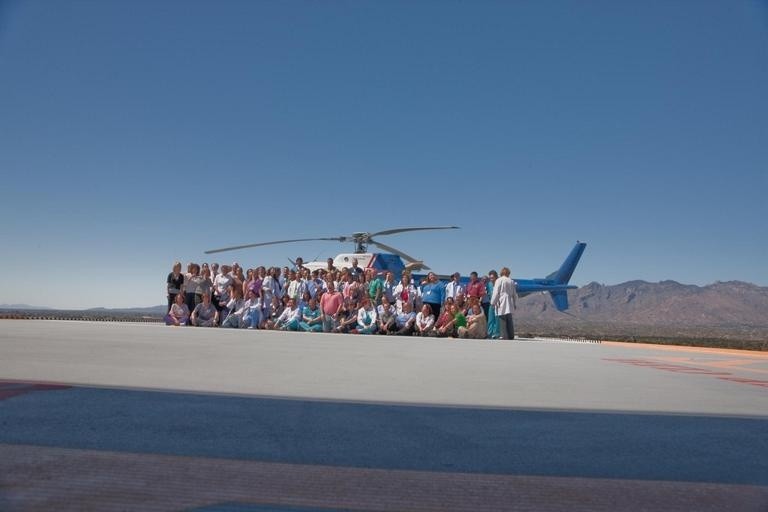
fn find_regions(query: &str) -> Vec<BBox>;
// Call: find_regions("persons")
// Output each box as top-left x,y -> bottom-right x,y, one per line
163,257 -> 516,340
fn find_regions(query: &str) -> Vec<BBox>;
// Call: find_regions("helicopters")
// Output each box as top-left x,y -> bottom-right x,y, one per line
204,225 -> 587,314
